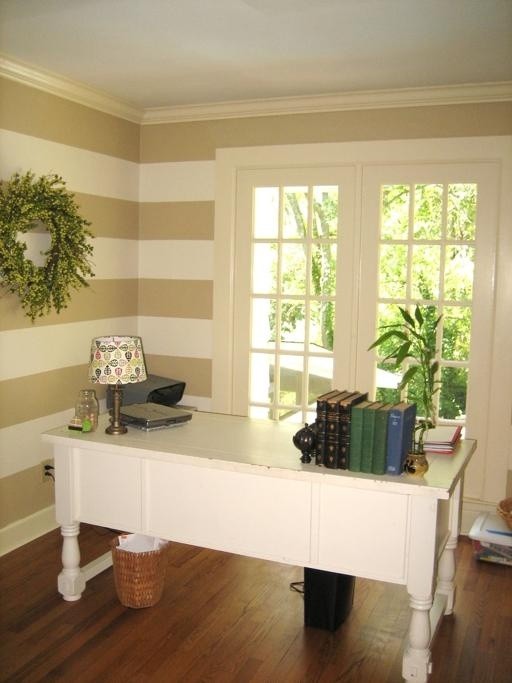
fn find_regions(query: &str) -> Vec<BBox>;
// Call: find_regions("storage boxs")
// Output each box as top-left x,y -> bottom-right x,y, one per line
468,512 -> 512,567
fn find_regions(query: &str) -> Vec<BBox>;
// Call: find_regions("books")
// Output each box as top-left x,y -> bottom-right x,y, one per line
313,388 -> 418,477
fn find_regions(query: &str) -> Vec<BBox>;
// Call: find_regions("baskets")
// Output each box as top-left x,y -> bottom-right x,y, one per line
112,536 -> 164,608
496,498 -> 512,530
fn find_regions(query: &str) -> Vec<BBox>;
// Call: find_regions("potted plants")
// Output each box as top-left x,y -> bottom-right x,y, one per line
365,304 -> 444,477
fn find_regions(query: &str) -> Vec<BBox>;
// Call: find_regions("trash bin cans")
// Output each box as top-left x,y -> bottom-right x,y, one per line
107,532 -> 169,610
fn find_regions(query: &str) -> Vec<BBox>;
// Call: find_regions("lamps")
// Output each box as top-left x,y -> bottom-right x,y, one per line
87,336 -> 147,436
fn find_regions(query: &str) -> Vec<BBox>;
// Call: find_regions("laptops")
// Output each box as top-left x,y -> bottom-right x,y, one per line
108,397 -> 193,429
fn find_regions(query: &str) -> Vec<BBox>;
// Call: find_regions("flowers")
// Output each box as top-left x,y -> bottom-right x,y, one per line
0,170 -> 96,326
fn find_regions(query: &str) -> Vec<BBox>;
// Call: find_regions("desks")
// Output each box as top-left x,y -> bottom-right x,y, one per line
39,403 -> 477,683
